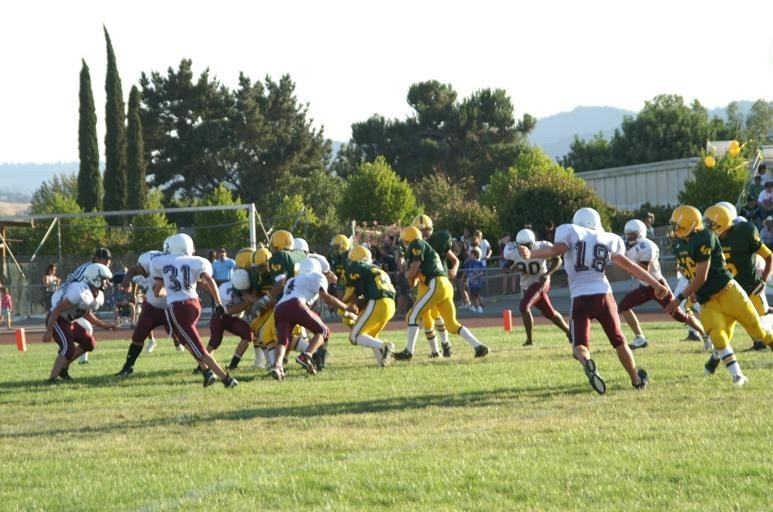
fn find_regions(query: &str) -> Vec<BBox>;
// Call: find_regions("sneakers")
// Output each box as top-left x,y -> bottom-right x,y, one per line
632,369 -> 648,392
629,338 -> 648,349
47,371 -> 71,384
731,375 -> 748,387
457,302 -> 483,313
680,331 -> 701,342
145,340 -> 156,352
176,344 -> 183,353
192,347 -> 326,388
375,328 -> 488,365
115,366 -> 132,377
567,334 -> 573,342
584,359 -> 606,395
746,342 -> 768,351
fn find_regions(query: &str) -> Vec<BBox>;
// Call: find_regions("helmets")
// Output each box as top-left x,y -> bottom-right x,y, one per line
348,246 -> 373,264
670,201 -> 739,240
515,228 -> 536,248
228,228 -> 330,293
624,219 -> 648,245
330,234 -> 349,251
399,226 -> 422,241
162,233 -> 194,257
83,263 -> 113,290
412,214 -> 433,230
572,207 -> 602,229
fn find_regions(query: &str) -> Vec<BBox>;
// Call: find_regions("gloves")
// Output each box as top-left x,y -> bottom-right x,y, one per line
213,305 -> 227,321
251,295 -> 271,313
750,279 -> 767,298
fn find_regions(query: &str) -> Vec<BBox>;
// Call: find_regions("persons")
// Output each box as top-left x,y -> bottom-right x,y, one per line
408,214 -> 460,358
392,226 -> 488,360
447,226 -> 491,312
642,162 -> 773,391
497,220 -> 557,295
51,248 -> 111,365
618,218 -> 711,350
519,207 -> 669,395
0,288 -> 12,329
333,217 -> 406,366
39,262 -> 117,387
150,233 -> 239,389
502,228 -> 572,346
112,229 -> 361,381
43,263 -> 61,327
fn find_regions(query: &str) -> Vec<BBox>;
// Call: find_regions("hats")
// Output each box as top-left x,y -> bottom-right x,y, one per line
746,195 -> 757,202
761,215 -> 773,227
95,247 -> 112,260
